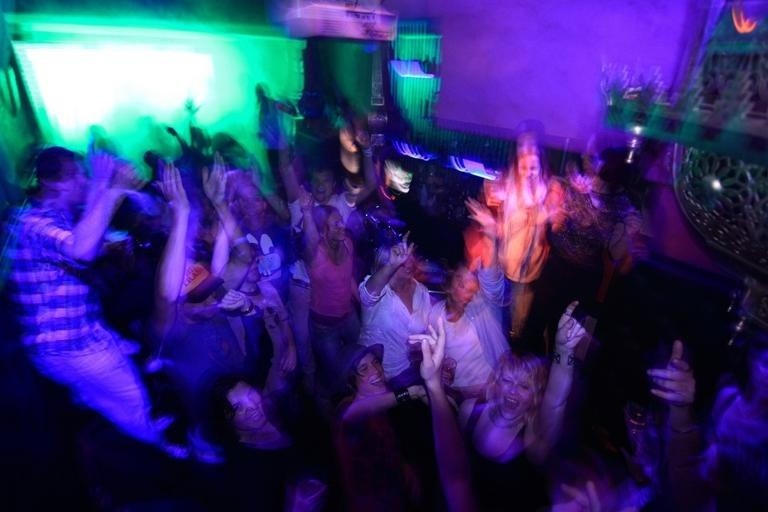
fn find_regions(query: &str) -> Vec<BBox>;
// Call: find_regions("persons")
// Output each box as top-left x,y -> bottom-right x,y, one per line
252,84 -> 296,191
0,118 -> 767,511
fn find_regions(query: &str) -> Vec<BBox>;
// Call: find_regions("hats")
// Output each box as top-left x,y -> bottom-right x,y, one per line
343,343 -> 384,374
177,257 -> 226,304
